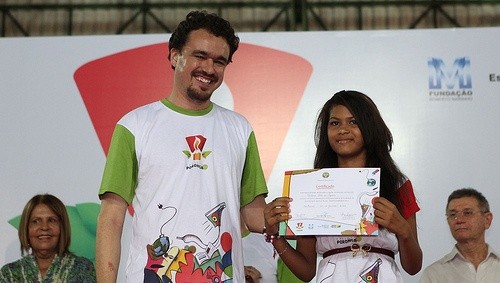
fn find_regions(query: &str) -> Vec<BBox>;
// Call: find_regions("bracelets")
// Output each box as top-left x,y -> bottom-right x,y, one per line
273,239 -> 288,259
263,226 -> 281,242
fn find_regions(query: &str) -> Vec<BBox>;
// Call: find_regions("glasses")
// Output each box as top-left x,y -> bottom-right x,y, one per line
445,210 -> 489,219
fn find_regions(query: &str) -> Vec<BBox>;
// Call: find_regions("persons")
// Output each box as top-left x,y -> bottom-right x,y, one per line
0,192 -> 97,283
418,188 -> 500,283
97,10 -> 269,283
263,91 -> 422,283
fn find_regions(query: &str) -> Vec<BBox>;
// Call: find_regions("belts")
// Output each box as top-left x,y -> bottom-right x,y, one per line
323,244 -> 394,260
